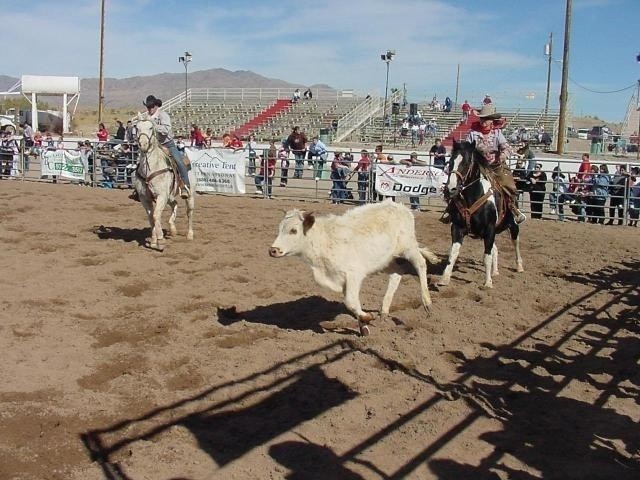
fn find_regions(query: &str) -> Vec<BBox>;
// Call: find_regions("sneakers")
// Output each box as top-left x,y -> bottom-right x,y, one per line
280,182 -> 287,186
255,190 -> 263,195
180,183 -> 191,200
550,211 -> 556,215
315,177 -> 320,181
307,165 -> 314,169
512,209 -> 525,224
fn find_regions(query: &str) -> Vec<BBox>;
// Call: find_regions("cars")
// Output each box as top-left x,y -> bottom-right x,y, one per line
568,124 -> 615,142
87,139 -> 137,178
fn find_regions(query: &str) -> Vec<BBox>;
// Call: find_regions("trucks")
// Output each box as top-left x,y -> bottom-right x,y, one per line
4,108 -> 72,133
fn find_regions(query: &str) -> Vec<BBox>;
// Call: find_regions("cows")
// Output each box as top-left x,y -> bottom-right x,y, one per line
268,196 -> 443,337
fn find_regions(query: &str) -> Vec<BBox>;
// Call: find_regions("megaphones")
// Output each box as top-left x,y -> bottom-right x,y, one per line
381,49 -> 395,61
179,51 -> 192,62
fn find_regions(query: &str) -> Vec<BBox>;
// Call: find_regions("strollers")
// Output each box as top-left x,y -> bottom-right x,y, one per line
329,167 -> 356,203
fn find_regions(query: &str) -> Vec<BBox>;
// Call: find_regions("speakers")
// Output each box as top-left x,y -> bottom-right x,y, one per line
392,103 -> 400,114
410,103 -> 417,115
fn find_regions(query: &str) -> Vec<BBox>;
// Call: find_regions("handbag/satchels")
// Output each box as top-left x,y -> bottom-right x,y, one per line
515,177 -> 534,192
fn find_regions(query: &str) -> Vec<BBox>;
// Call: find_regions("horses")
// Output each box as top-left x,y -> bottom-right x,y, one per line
437,136 -> 524,293
129,111 -> 197,251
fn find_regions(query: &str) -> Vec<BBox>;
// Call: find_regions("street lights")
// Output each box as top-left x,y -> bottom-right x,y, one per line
178,51 -> 192,136
379,49 -> 396,150
544,30 -> 554,115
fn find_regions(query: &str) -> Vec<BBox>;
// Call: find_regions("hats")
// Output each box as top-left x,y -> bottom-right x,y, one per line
123,140 -> 129,146
143,95 -> 162,108
473,104 -> 501,120
388,154 -> 393,158
377,154 -> 385,159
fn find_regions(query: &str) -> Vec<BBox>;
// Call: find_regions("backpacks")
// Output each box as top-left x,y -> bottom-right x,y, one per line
602,175 -> 614,194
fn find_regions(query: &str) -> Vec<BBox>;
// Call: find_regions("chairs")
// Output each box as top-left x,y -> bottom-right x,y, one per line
167,97 -> 250,143
498,111 -> 559,148
364,108 -> 467,154
250,95 -> 381,147
